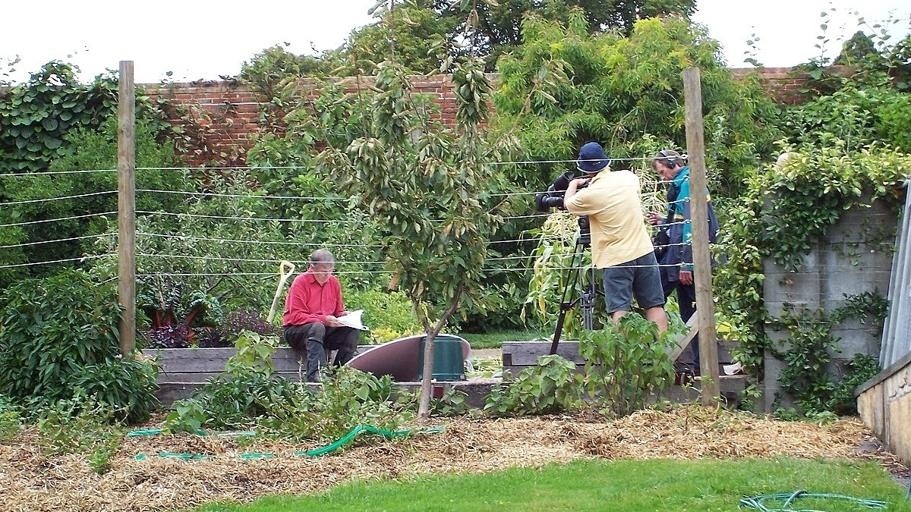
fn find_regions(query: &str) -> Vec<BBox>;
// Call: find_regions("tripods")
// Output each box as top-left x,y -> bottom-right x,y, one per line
543,216 -> 602,363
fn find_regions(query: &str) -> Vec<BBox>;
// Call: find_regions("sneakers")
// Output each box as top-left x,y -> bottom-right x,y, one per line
308,372 -> 323,383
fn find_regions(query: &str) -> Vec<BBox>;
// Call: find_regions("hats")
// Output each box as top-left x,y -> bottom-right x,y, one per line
576,142 -> 611,173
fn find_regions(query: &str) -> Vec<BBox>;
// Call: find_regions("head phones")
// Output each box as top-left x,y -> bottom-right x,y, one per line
660,150 -> 677,169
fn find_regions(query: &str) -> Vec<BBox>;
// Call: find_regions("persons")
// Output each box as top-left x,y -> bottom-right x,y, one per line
564,140 -> 669,350
644,149 -> 720,378
282,248 -> 359,383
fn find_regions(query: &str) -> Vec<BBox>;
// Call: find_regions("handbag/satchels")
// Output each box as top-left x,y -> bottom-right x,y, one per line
653,231 -> 670,259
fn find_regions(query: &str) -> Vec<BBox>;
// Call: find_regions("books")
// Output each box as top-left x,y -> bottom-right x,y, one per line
333,309 -> 369,331
